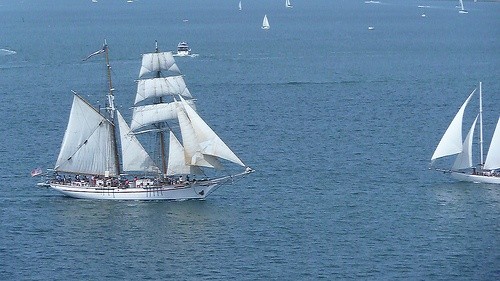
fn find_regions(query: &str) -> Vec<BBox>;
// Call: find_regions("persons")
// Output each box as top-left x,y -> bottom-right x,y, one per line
470,165 -> 500,178
46,173 -> 209,192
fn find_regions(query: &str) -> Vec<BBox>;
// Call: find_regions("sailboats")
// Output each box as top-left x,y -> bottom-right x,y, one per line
456,0 -> 468,14
429,82 -> 500,184
238,1 -> 243,11
260,14 -> 271,31
285,0 -> 293,9
30,37 -> 256,200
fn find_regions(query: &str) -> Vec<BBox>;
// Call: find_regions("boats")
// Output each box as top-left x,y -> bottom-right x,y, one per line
369,25 -> 375,30
364,0 -> 380,4
154,40 -> 199,58
